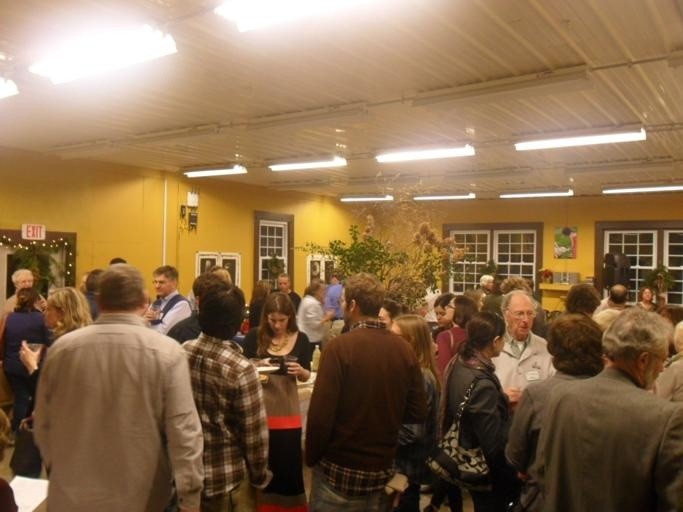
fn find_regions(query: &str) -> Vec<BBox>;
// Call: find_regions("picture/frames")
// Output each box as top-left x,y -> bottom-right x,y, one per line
553,227 -> 577,259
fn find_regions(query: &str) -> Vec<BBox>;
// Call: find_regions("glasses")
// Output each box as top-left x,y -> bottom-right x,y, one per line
152,280 -> 167,285
510,309 -> 536,319
447,303 -> 454,310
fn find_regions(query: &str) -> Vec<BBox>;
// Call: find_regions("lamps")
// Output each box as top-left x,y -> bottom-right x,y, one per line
498,187 -> 574,197
512,122 -> 647,151
183,163 -> 249,178
412,192 -> 476,200
246,102 -> 368,131
129,124 -> 219,144
47,138 -> 112,153
270,156 -> 348,171
340,194 -> 394,201
600,183 -> 683,195
374,140 -> 479,164
411,65 -> 592,112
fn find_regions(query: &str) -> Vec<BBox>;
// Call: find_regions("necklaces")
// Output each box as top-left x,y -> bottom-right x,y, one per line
267,332 -> 289,352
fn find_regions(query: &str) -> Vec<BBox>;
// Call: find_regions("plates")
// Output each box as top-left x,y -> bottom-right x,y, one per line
256,366 -> 280,374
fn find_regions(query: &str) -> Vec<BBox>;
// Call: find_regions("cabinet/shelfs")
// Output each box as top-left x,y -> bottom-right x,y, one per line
539,283 -> 594,310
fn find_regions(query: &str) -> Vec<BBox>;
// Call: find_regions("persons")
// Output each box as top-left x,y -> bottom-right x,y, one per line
181,281 -> 274,508
531,308 -> 683,510
148,265 -> 192,340
243,291 -> 313,512
249,280 -> 271,325
435,312 -> 522,511
21,287 -> 93,379
482,277 -> 506,314
388,315 -> 442,511
505,313 -> 603,475
274,274 -> 300,308
655,319 -> 683,401
0,270 -> 46,315
209,266 -> 232,287
33,263 -> 204,511
633,287 -> 657,312
593,283 -> 630,329
558,226 -> 577,259
378,300 -> 407,328
491,290 -> 556,404
167,273 -> 226,342
83,268 -> 104,320
462,288 -> 484,314
475,274 -> 493,306
325,274 -> 342,318
432,292 -> 455,340
0,289 -> 44,427
562,283 -> 601,317
435,297 -> 479,370
292,279 -> 336,346
306,272 -> 427,511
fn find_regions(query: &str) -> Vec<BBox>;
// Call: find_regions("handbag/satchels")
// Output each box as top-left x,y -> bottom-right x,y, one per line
426,374 -> 497,493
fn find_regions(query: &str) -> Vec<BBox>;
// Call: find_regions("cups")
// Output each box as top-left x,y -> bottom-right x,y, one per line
150,305 -> 161,320
284,355 -> 298,372
26,343 -> 42,364
